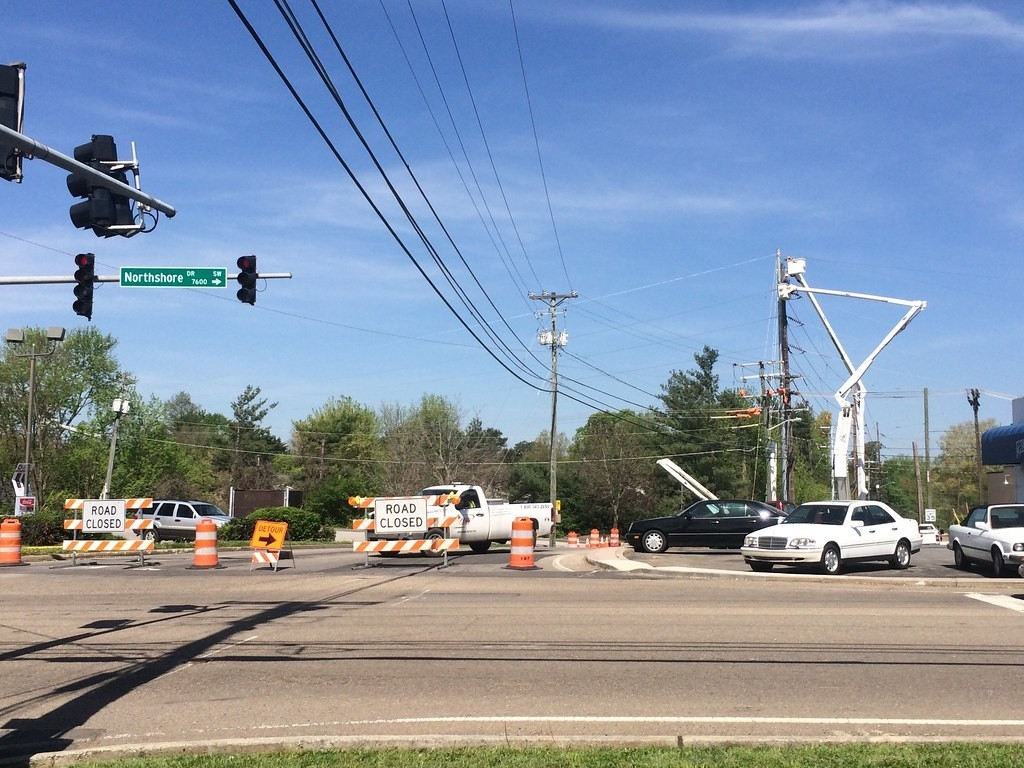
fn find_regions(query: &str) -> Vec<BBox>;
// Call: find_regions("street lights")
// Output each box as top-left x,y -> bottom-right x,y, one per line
7,323 -> 67,519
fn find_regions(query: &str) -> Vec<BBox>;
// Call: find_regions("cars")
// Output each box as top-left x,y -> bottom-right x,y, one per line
946,502 -> 1024,578
625,499 -> 807,554
919,523 -> 944,546
741,500 -> 924,576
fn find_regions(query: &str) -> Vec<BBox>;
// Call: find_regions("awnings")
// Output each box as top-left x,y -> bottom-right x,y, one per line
982,424 -> 1024,465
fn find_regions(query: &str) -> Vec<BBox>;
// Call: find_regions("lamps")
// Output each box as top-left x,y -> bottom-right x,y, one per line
1003,474 -> 1011,485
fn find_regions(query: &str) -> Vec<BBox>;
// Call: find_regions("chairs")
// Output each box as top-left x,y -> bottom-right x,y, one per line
820,513 -> 835,521
853,512 -> 865,523
991,515 -> 1001,528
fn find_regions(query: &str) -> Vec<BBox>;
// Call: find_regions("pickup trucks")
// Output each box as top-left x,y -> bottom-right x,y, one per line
367,481 -> 554,556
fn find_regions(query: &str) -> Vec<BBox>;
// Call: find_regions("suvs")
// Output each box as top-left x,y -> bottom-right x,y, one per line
132,498 -> 240,542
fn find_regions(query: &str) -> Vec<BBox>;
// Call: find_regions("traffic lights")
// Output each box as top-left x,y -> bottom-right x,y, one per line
66,132 -> 135,240
237,255 -> 256,306
72,253 -> 95,318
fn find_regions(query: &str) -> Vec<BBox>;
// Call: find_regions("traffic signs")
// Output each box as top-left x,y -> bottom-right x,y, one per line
118,265 -> 228,290
